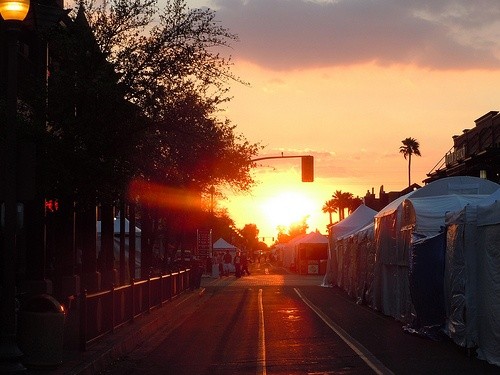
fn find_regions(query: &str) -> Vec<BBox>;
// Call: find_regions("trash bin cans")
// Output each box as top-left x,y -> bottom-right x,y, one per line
190,265 -> 203,290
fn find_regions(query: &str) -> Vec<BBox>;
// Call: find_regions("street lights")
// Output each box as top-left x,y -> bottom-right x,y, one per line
208,185 -> 214,256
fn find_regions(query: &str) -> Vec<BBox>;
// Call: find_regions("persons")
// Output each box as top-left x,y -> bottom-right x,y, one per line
162,244 -> 281,279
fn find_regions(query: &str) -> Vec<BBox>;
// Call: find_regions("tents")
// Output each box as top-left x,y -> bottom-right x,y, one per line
329,176 -> 499,368
212,237 -> 240,275
281,231 -> 327,276
